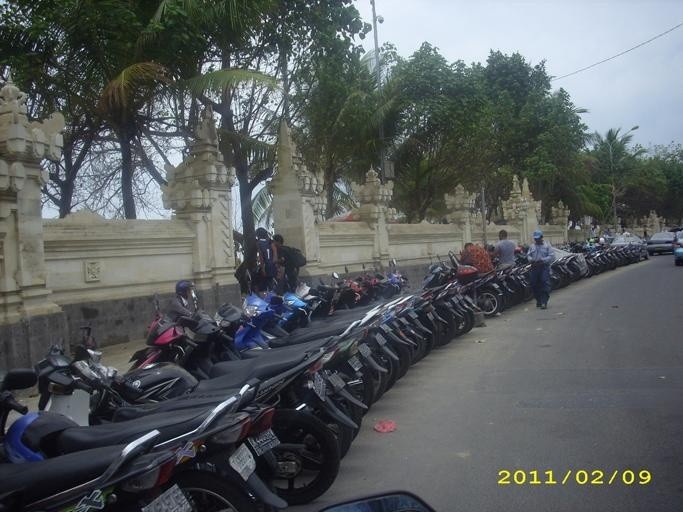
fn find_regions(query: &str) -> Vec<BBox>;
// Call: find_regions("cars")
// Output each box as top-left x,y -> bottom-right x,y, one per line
647,223 -> 683,266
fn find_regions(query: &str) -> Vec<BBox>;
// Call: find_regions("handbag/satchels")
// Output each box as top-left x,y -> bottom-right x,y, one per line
290,247 -> 306,267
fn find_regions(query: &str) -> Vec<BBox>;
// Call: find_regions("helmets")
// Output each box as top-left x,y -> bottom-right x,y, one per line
176,280 -> 194,296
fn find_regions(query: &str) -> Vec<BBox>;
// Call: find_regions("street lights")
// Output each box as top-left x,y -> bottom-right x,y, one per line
610,124 -> 642,233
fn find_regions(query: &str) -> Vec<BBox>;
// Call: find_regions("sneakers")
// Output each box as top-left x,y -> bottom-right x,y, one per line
536,303 -> 546,308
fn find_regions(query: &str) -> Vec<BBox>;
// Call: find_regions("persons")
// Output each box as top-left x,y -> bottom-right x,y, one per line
526,230 -> 555,310
488,229 -> 517,270
165,280 -> 198,323
254,226 -> 280,291
457,242 -> 492,274
235,232 -> 259,291
270,232 -> 302,289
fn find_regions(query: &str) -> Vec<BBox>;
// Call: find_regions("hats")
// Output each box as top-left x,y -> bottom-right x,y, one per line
533,231 -> 542,239
